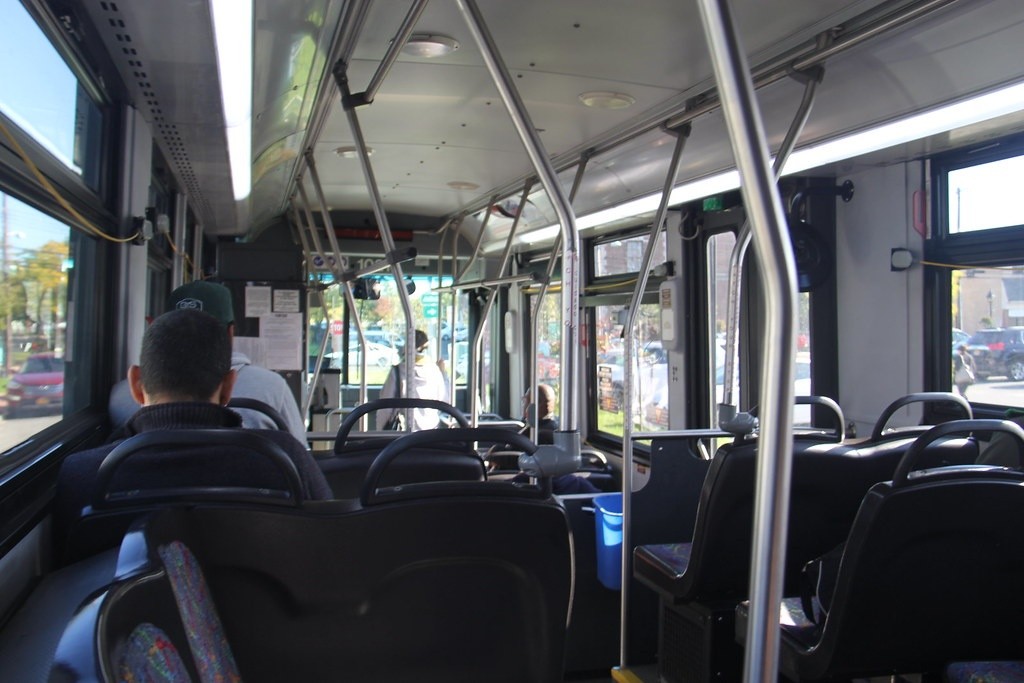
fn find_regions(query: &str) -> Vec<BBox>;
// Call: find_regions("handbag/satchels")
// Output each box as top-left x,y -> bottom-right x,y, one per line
383,365 -> 406,432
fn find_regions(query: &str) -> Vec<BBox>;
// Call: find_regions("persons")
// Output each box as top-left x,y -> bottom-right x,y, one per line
488,385 -> 558,471
55,309 -> 333,561
350,282 -> 380,299
376,330 -> 445,431
109,280 -> 306,446
954,346 -> 975,399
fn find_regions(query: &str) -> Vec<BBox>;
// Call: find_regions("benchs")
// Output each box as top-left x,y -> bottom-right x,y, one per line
0,392 -> 1024,683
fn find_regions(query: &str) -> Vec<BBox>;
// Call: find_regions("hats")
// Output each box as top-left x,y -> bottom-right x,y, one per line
167,279 -> 234,325
